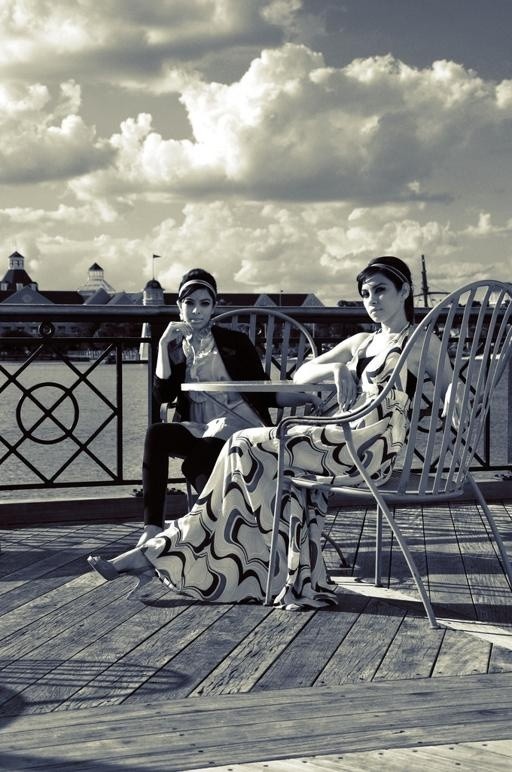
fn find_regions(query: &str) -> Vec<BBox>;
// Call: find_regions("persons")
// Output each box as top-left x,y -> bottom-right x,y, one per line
135,267 -> 274,547
86,256 -> 473,614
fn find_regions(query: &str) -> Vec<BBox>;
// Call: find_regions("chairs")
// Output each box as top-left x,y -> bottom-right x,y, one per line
158,305 -> 318,517
260,280 -> 512,631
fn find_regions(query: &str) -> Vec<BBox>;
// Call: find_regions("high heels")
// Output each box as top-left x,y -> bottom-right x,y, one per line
87,556 -> 155,599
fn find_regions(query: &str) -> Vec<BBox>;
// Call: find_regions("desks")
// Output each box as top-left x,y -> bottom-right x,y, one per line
180,379 -> 337,392
